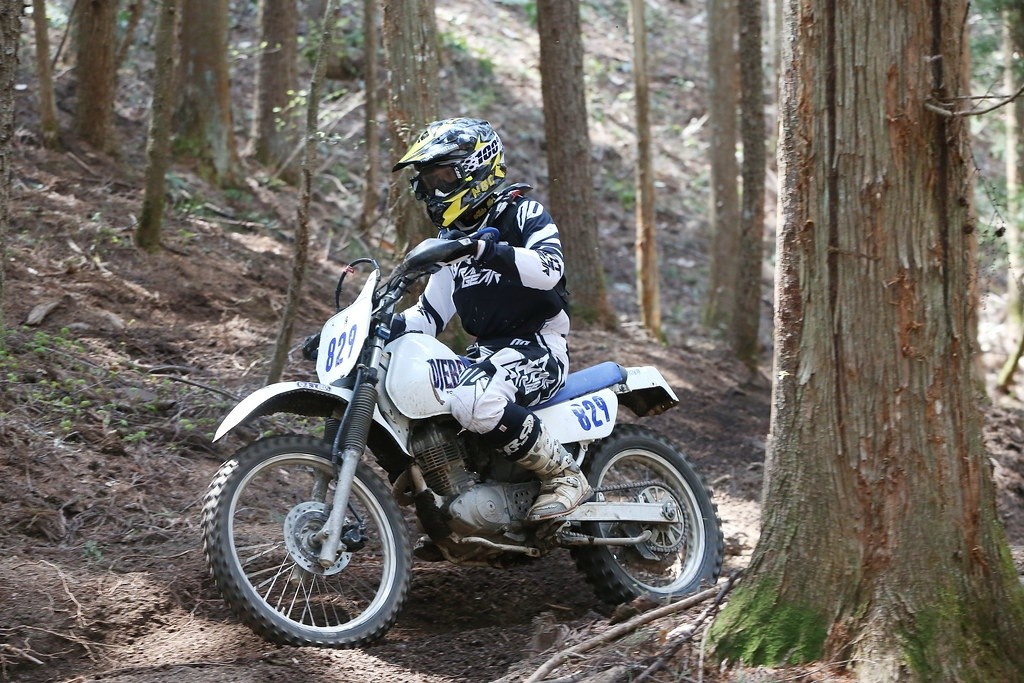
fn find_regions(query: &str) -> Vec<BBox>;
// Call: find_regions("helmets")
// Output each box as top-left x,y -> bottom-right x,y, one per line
392,118 -> 508,232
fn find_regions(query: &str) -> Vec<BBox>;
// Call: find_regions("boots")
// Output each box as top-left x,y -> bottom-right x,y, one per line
494,407 -> 594,521
412,535 -> 447,562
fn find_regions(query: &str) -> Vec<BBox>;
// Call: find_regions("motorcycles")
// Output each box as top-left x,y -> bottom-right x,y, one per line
199,239 -> 724,649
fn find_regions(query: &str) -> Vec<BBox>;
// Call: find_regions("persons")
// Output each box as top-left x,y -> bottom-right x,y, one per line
302,115 -> 595,562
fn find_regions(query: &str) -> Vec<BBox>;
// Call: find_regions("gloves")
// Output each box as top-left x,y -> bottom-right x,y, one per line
433,236 -> 478,268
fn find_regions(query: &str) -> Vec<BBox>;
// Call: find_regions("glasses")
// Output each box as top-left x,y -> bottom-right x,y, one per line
409,163 -> 467,201
